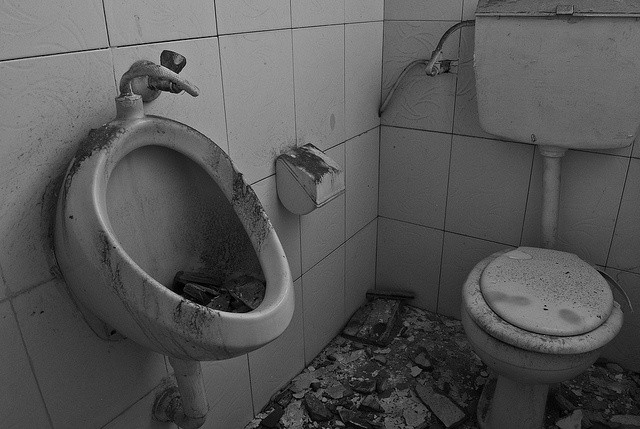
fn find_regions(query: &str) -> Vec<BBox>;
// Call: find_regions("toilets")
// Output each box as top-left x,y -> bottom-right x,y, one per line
461,1 -> 640,429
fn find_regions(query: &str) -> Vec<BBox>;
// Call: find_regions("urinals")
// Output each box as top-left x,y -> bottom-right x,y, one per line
54,50 -> 295,429
